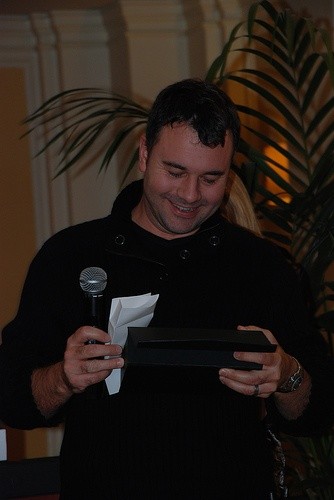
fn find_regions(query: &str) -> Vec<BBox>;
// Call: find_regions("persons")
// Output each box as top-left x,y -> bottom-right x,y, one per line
0,79 -> 334,500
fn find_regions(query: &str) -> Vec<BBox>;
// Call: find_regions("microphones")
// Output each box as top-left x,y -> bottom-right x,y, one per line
79,267 -> 107,360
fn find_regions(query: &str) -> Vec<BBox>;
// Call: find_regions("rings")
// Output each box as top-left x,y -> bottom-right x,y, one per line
255,385 -> 259,395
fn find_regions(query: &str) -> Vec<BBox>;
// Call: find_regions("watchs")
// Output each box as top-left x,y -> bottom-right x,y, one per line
276,357 -> 303,394
